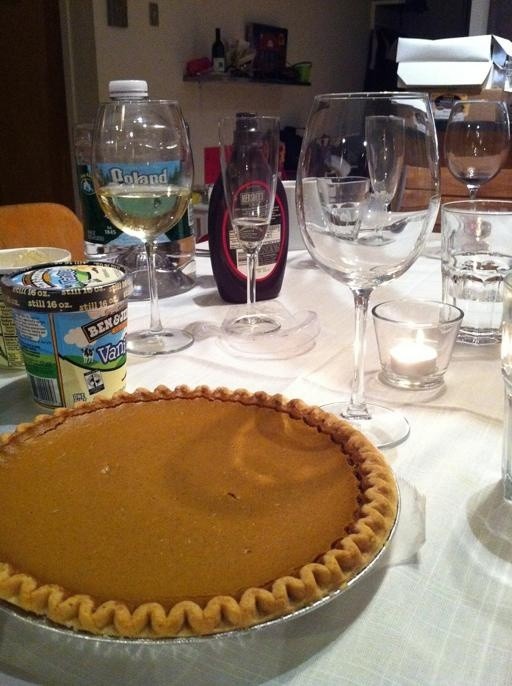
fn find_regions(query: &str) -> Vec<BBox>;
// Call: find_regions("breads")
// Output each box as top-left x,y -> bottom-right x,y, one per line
0,384 -> 399,638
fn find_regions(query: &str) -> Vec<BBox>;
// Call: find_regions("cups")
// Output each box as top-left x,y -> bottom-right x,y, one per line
0,247 -> 131,412
293,63 -> 312,85
280,180 -> 310,251
372,200 -> 512,404
362,114 -> 405,209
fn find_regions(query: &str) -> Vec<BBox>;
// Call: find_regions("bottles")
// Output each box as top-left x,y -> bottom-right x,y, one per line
211,28 -> 225,72
207,112 -> 289,304
72,80 -> 196,294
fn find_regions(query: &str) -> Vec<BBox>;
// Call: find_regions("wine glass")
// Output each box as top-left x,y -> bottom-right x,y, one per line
294,91 -> 441,448
444,100 -> 511,201
218,116 -> 282,337
92,98 -> 195,355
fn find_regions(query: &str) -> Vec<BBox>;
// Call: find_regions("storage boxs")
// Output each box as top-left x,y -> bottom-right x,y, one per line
392,41 -> 512,218
